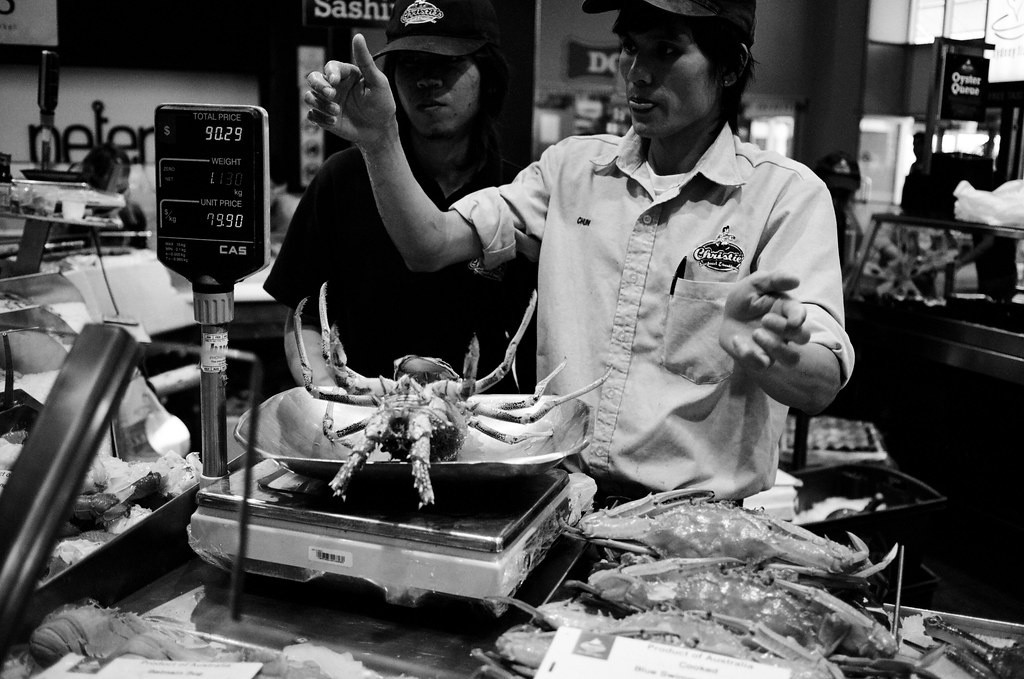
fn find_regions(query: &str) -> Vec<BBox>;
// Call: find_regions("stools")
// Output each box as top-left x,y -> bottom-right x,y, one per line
778,412 -> 889,471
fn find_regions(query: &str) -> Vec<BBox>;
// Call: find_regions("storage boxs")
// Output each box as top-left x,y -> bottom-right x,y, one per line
787,461 -> 952,607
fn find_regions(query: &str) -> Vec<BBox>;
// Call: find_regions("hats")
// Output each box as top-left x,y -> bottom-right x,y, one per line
581,0 -> 756,38
370,0 -> 497,61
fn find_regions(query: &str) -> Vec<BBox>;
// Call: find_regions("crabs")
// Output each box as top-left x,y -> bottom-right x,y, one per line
557,495 -> 900,577
292,278 -> 615,510
563,553 -> 948,671
470,592 -> 943,679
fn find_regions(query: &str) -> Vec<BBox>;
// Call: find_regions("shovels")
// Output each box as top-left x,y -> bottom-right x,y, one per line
824,493 -> 885,520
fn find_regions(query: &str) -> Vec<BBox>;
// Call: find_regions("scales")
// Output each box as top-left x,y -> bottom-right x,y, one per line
155,103 -> 572,618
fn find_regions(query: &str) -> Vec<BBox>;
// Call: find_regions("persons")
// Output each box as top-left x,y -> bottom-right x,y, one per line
66,142 -> 148,250
814,148 -> 864,272
302,0 -> 857,510
262,0 -> 542,394
901,132 -> 934,210
